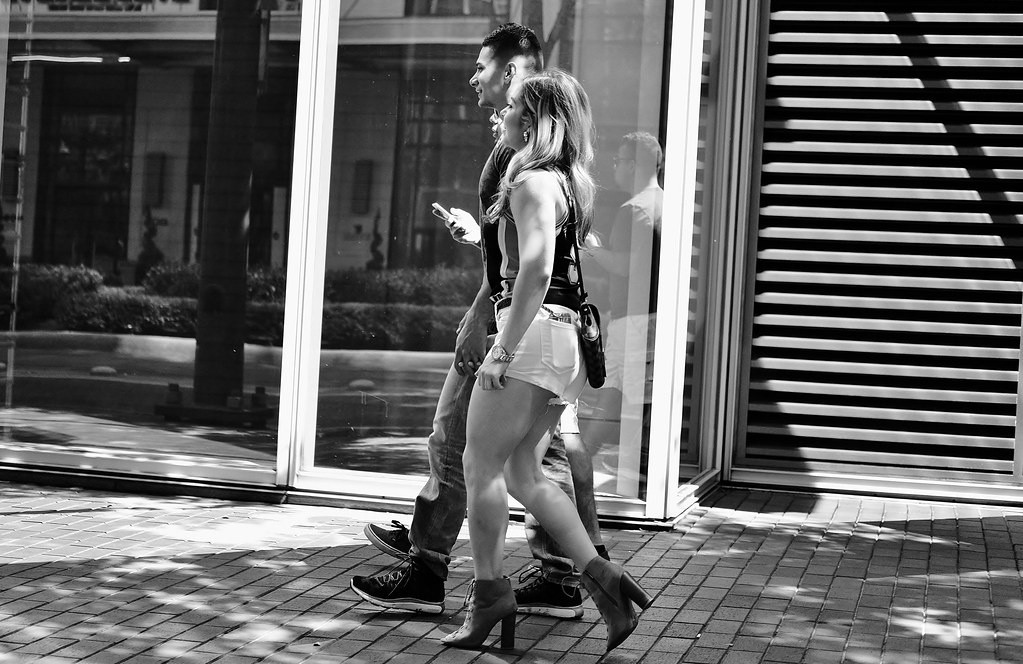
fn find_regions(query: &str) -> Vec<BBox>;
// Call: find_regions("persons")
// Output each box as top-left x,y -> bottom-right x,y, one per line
432,64 -> 654,653
580,129 -> 664,484
351,22 -> 614,620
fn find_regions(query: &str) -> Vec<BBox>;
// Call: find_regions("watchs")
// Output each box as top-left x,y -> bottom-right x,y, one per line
491,344 -> 515,363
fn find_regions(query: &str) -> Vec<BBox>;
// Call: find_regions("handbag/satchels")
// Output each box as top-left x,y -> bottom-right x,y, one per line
580,306 -> 607,388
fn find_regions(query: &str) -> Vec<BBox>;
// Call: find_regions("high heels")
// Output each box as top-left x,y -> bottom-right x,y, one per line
580,556 -> 654,651
441,576 -> 518,651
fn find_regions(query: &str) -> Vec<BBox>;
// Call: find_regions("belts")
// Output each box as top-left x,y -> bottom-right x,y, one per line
497,293 -> 578,315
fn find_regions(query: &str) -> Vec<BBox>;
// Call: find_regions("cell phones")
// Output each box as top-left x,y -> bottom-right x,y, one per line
432,202 -> 473,235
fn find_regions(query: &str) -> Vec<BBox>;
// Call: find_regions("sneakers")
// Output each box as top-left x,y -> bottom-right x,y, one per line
513,566 -> 584,618
350,555 -> 445,613
571,543 -> 610,574
364,520 -> 415,563
